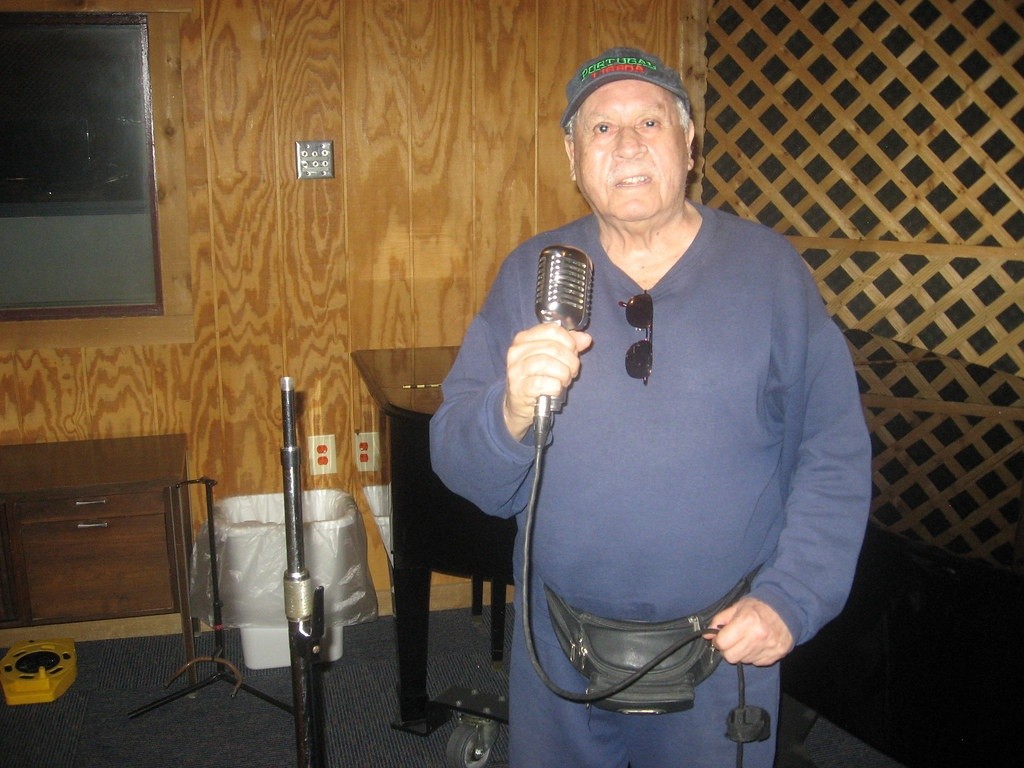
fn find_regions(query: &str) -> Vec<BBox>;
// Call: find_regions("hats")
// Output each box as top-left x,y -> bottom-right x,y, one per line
560,47 -> 691,133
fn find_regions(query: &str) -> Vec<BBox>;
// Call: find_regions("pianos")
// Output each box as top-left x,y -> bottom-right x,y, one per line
350,344 -> 520,739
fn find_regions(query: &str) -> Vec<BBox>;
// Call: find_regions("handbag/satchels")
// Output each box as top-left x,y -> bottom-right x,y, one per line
545,563 -> 766,716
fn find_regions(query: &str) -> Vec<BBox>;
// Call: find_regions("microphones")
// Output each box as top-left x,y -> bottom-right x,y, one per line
533,245 -> 593,447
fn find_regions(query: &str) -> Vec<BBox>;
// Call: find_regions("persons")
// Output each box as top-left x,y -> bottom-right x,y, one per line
428,45 -> 871,768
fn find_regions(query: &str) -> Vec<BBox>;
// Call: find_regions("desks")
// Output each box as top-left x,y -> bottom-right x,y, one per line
350,345 -> 518,737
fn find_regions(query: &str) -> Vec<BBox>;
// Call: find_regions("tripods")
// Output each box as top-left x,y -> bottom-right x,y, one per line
125,478 -> 295,721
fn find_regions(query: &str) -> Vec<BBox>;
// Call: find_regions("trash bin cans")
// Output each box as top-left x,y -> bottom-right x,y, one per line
214,490 -> 356,672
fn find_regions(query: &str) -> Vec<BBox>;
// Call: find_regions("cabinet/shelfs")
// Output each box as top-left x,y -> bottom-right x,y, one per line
0,435 -> 202,699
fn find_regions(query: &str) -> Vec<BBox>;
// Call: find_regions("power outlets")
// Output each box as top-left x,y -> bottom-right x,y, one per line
355,432 -> 374,472
308,434 -> 337,476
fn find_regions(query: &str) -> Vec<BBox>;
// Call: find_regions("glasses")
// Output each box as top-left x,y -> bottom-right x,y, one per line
618,290 -> 654,386
